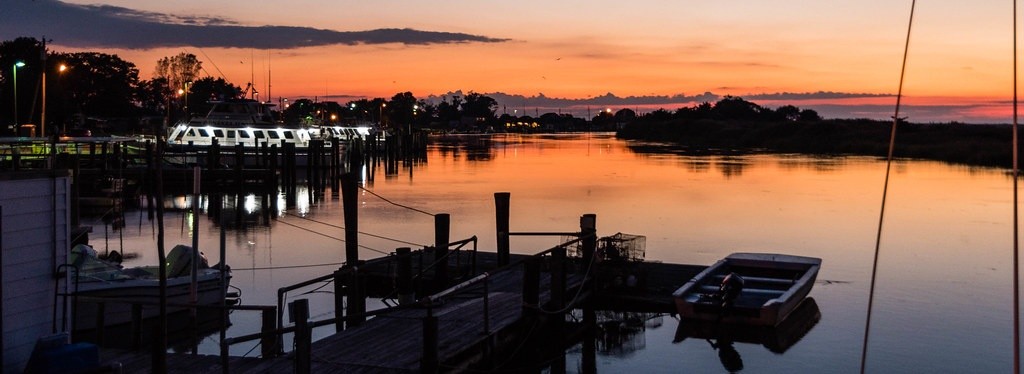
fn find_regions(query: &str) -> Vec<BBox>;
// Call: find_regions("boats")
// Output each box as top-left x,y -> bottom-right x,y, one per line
110,46 -> 394,169
671,249 -> 823,329
70,224 -> 232,330
673,295 -> 823,374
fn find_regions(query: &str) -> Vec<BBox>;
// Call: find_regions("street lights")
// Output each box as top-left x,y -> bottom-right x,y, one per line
282,97 -> 288,113
379,102 -> 386,127
252,85 -> 259,99
317,101 -> 327,127
178,81 -> 189,122
39,62 -> 69,138
12,59 -> 26,135
